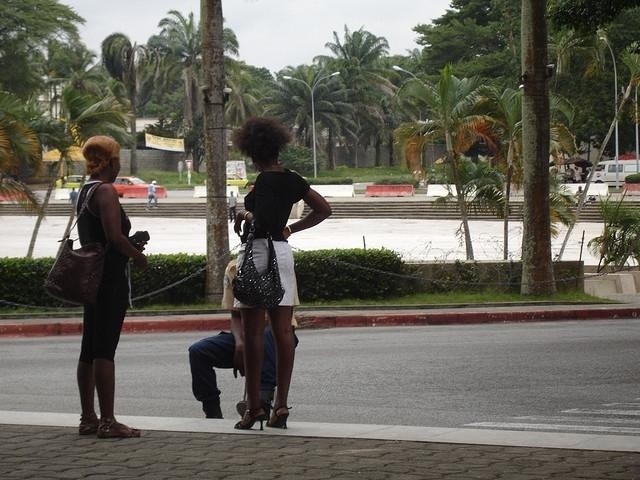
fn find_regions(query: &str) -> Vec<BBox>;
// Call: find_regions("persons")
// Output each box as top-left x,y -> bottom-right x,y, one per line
573,186 -> 584,206
551,164 -> 589,184
188,238 -> 300,418
232,116 -> 332,431
227,193 -> 236,224
147,179 -> 159,208
77,134 -> 147,438
69,187 -> 78,215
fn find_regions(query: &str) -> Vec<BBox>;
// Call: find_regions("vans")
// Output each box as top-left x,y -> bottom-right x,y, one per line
585,160 -> 640,184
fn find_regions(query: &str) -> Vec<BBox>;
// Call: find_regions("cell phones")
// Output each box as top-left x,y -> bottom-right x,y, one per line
130,231 -> 150,242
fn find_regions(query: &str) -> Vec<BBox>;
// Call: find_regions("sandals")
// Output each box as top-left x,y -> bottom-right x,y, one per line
78,412 -> 141,438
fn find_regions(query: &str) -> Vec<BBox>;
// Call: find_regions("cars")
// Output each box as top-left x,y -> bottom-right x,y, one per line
54,173 -> 91,189
112,175 -> 168,198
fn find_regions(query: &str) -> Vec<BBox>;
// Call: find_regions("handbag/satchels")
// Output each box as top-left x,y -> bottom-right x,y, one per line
43,239 -> 105,307
232,220 -> 285,309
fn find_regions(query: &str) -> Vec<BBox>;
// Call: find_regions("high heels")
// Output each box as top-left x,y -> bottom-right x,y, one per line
266,405 -> 292,429
234,405 -> 265,430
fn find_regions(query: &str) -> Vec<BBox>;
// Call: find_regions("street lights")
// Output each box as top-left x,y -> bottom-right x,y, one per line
547,64 -> 556,70
282,71 -> 341,179
598,36 -> 621,191
389,63 -> 428,169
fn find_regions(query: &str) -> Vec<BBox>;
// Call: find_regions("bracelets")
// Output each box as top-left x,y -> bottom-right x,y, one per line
286,225 -> 292,235
244,211 -> 250,222
